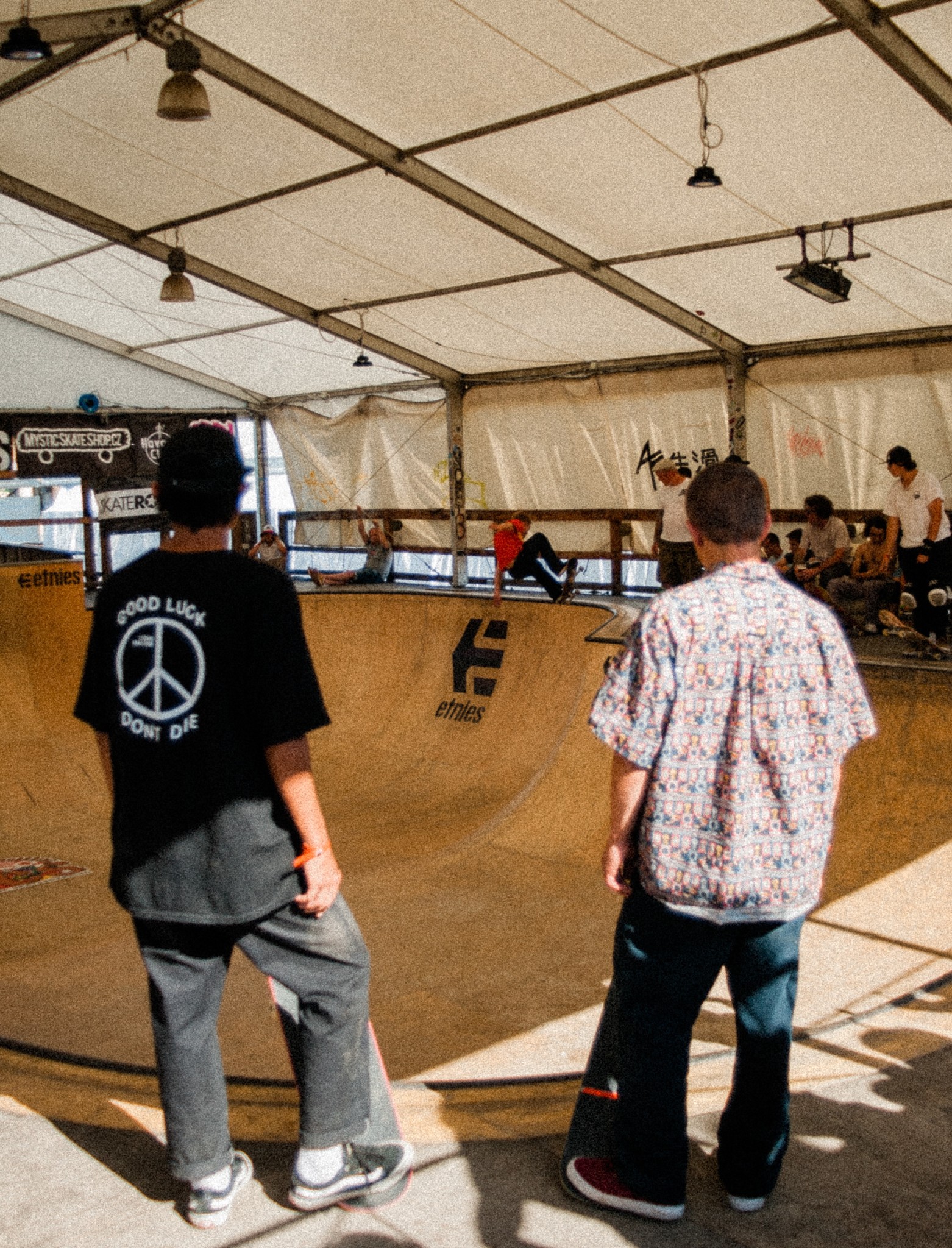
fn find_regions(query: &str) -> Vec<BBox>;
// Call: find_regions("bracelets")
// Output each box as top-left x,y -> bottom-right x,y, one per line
884,553 -> 893,561
292,837 -> 331,868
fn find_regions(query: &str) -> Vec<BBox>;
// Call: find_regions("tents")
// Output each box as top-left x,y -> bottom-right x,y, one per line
2,2 -> 951,595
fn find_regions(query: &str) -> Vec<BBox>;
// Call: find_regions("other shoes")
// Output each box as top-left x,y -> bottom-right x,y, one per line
903,646 -> 919,657
940,645 -> 951,652
289,1140 -> 416,1212
308,566 -> 325,586
567,1157 -> 685,1219
555,591 -> 564,603
729,1193 -> 765,1210
556,560 -> 569,577
187,1152 -> 253,1228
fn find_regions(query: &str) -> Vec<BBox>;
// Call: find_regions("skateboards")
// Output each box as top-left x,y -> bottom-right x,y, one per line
878,610 -> 952,661
560,556 -> 584,606
814,586 -> 866,636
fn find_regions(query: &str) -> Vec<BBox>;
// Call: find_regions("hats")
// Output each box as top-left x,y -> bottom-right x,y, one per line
159,422 -> 256,483
260,525 -> 277,536
725,454 -> 750,465
652,457 -> 674,472
879,445 -> 911,464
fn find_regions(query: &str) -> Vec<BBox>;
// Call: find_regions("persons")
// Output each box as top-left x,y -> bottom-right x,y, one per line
724,454 -> 750,467
72,422 -> 412,1229
772,528 -> 816,592
793,495 -> 850,600
560,464 -> 876,1223
247,525 -> 288,573
878,446 -> 952,651
846,524 -> 868,545
652,459 -> 703,592
763,532 -> 790,566
308,506 -> 393,587
487,511 -> 576,609
827,515 -> 898,631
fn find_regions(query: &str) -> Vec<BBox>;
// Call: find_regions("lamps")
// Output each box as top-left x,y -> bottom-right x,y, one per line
155,36 -> 213,122
354,352 -> 372,367
159,248 -> 196,303
0,0 -> 53,61
687,152 -> 722,188
783,261 -> 854,304
